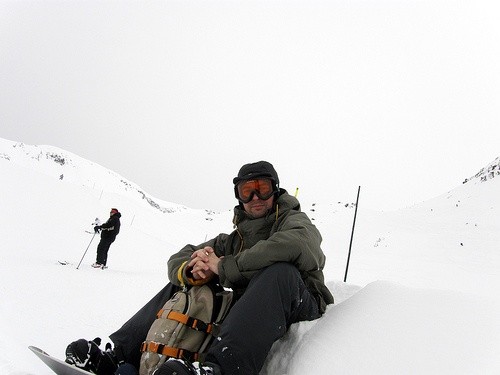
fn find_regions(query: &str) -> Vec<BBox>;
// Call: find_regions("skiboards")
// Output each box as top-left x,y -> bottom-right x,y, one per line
57,259 -> 108,270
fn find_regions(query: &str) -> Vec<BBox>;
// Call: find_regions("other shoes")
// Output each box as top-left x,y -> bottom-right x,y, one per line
157,359 -> 198,375
64,339 -> 118,375
92,263 -> 104,269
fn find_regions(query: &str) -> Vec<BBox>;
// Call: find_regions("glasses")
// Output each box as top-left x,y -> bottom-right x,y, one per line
234,177 -> 278,204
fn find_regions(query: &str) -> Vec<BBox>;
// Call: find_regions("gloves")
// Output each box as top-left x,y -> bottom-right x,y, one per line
94,225 -> 101,233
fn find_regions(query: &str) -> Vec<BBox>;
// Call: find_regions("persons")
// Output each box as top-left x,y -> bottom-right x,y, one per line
91,208 -> 121,268
65,162 -> 335,375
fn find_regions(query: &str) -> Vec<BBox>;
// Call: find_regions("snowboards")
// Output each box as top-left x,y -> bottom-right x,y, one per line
27,345 -> 96,375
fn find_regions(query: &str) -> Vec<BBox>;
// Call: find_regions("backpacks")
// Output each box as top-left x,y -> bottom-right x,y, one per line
139,280 -> 234,375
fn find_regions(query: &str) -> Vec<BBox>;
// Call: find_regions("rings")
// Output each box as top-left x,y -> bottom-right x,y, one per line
205,252 -> 208,255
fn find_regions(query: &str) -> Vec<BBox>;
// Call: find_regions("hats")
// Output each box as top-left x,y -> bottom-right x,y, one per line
233,161 -> 280,184
